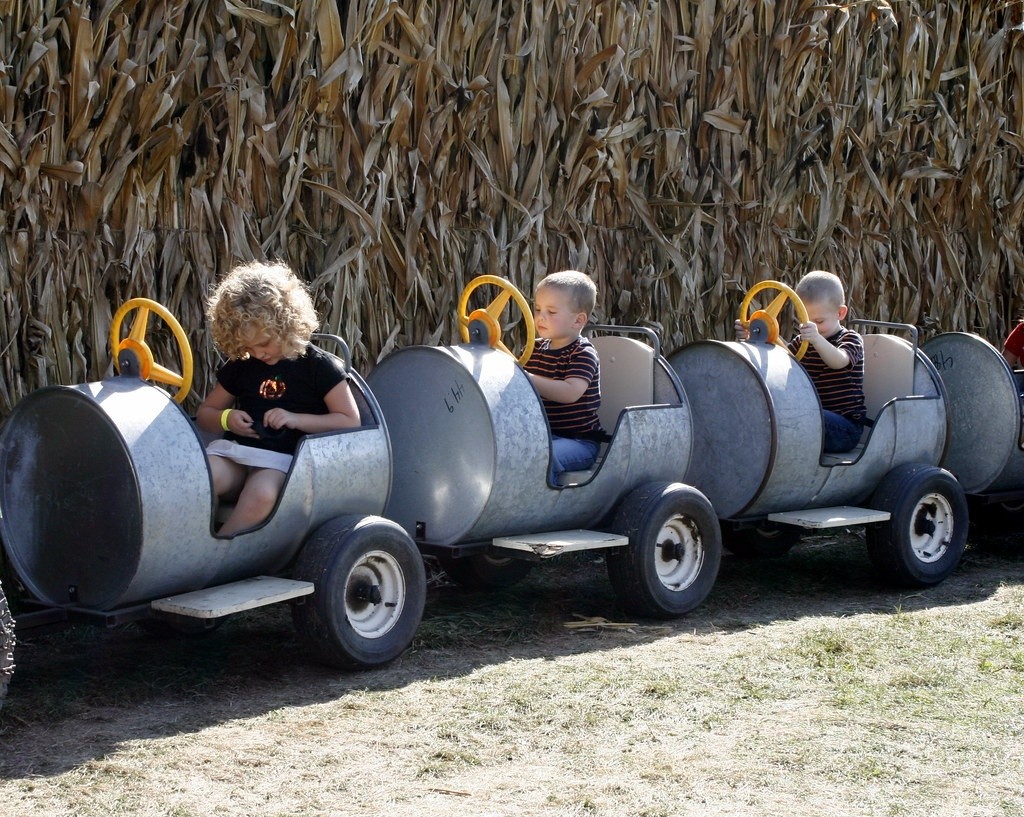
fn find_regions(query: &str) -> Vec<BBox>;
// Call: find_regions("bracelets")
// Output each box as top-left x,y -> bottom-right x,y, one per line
220,409 -> 232,431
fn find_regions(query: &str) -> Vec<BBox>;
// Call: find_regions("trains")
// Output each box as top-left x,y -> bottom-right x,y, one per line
2,272 -> 1022,676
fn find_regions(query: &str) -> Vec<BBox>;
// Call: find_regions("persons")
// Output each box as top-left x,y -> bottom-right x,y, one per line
197,258 -> 361,536
1000,321 -> 1024,451
734,270 -> 867,454
511,270 -> 606,488
